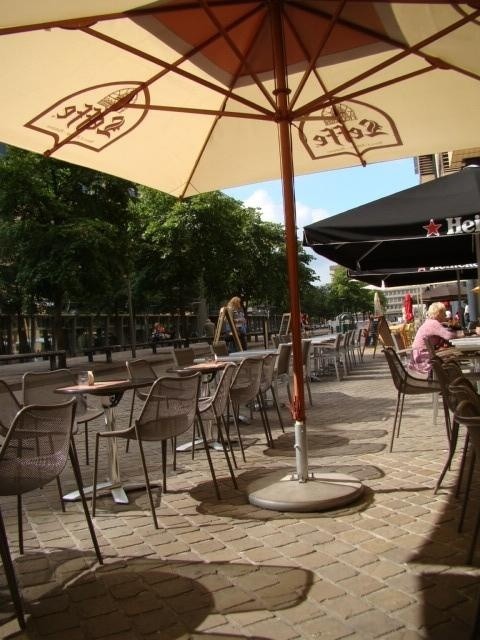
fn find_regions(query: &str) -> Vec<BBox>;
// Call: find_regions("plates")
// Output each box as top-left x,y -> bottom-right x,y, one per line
449,338 -> 480,346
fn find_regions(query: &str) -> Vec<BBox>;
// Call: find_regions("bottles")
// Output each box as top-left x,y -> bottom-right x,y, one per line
87,370 -> 95,386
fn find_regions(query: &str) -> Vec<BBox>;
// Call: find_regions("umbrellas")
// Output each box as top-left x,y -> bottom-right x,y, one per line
0,0 -> 480,483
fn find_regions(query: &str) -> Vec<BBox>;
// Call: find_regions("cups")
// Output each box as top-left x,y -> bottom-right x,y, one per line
204,354 -> 218,365
77,371 -> 88,386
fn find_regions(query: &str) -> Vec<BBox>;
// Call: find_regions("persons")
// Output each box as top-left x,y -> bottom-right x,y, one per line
408,302 -> 464,373
225,297 -> 247,353
445,300 -> 469,324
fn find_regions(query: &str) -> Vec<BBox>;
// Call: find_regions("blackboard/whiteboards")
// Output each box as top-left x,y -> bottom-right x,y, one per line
364,316 -> 383,348
278,314 -> 291,337
